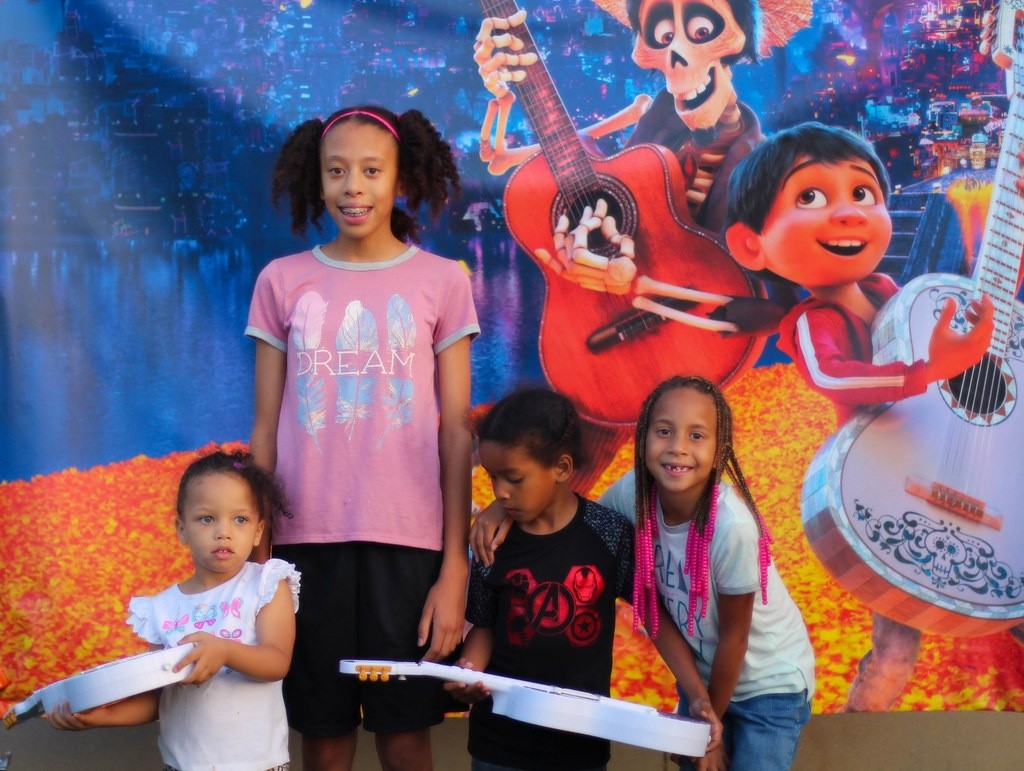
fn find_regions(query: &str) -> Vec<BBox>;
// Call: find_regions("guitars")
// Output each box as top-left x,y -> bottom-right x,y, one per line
339,660 -> 711,757
2,642 -> 197,731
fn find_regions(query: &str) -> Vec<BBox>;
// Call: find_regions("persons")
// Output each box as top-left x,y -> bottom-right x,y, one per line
38,451 -> 302,771
443,389 -> 724,770
470,375 -> 816,771
244,106 -> 482,771
